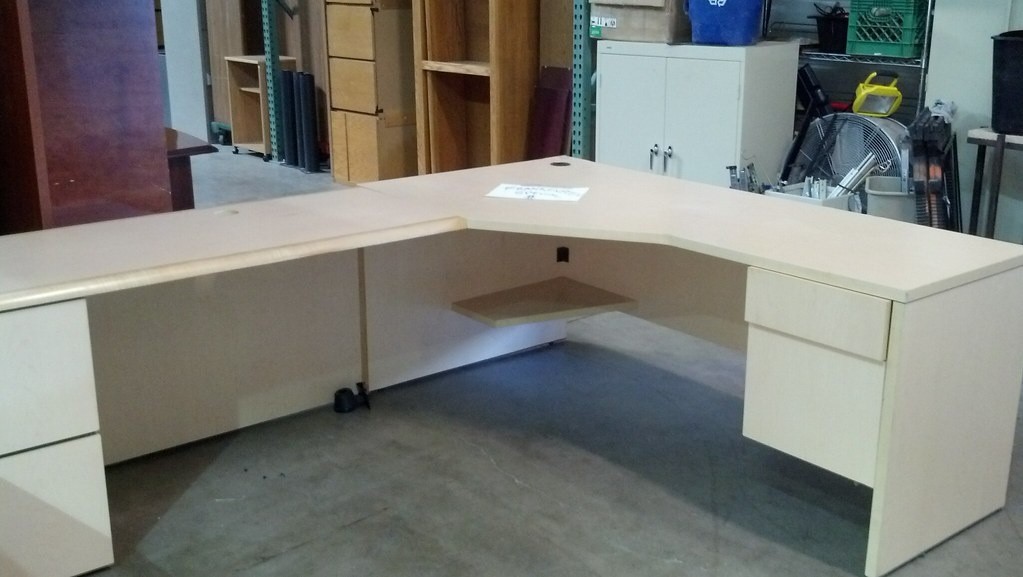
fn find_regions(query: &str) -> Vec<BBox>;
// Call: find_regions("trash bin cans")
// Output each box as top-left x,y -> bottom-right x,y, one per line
990,30 -> 1023,136
865,176 -> 915,224
682,0 -> 765,46
765,181 -> 854,212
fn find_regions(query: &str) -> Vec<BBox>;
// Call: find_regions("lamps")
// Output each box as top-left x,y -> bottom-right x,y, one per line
853,70 -> 903,117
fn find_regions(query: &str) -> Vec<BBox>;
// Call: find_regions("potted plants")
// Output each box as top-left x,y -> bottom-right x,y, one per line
807,2 -> 849,54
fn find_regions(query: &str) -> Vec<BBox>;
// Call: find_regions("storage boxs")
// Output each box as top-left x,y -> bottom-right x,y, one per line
763,182 -> 849,211
865,176 -> 919,224
846,0 -> 930,59
589,0 -> 692,44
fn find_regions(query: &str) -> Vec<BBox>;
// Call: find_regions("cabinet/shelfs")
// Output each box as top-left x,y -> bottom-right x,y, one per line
0,298 -> 115,577
762,0 -> 934,117
206,0 -> 572,186
595,39 -> 800,193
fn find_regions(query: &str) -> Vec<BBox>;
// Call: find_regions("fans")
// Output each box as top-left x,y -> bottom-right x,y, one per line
777,113 -> 913,210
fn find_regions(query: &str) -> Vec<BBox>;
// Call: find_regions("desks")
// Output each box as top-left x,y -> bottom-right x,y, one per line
164,128 -> 218,210
0,155 -> 1023,577
966,127 -> 1023,239
